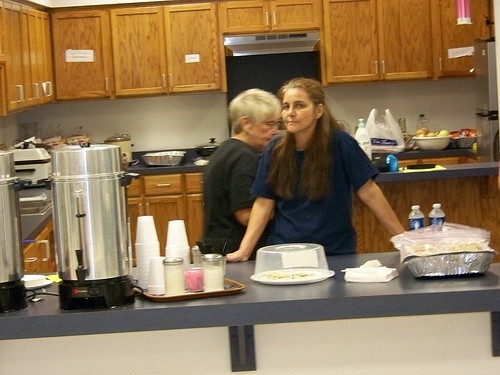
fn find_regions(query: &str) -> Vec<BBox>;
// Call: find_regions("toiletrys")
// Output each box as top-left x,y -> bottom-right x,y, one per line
354,118 -> 372,162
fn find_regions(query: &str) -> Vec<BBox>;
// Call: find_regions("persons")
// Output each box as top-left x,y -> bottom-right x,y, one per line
225,77 -> 406,263
194,89 -> 283,263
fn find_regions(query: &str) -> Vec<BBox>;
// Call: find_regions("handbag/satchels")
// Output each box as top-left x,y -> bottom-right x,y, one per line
365,109 -> 406,154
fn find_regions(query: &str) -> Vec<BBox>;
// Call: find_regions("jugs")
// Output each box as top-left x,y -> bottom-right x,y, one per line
47,141 -> 140,311
0,150 -> 29,314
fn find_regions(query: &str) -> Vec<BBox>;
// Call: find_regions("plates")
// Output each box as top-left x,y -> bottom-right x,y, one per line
249,269 -> 335,286
24,280 -> 54,289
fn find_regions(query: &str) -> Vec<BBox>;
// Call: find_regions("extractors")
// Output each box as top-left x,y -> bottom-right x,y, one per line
224,31 -> 320,57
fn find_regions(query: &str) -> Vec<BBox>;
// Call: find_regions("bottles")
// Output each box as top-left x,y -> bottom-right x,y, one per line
416,114 -> 427,130
407,204 -> 425,231
354,118 -> 372,162
428,203 -> 445,226
162,257 -> 185,295
200,253 -> 225,292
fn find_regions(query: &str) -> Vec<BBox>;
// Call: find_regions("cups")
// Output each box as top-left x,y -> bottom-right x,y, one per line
134,215 -> 191,296
185,267 -> 203,291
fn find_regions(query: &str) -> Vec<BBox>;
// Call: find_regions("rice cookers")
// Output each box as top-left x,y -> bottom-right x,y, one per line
104,133 -> 133,165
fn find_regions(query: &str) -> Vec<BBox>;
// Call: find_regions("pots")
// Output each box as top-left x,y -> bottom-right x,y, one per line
193,137 -> 221,157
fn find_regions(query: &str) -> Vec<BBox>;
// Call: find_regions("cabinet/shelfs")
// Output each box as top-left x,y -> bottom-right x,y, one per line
218,0 -> 322,34
0,0 -> 56,117
23,222 -> 55,273
108,2 -> 228,102
126,172 -> 205,256
431,0 -> 494,81
319,0 -> 432,87
50,7 -> 112,101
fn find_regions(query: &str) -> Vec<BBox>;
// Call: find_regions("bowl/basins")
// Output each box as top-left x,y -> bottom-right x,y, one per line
451,138 -> 476,149
412,136 -> 451,151
